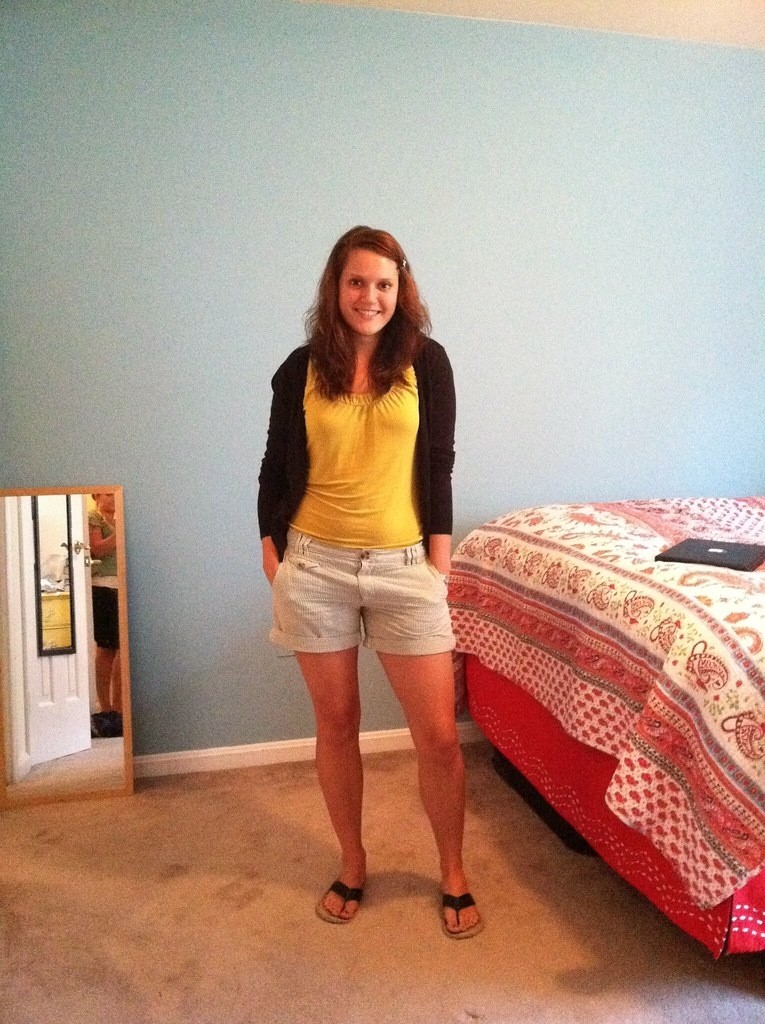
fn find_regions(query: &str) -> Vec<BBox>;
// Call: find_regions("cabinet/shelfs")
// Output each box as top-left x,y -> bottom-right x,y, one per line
41,590 -> 71,649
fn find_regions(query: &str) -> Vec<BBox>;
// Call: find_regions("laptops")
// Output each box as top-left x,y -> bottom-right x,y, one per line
653,537 -> 765,572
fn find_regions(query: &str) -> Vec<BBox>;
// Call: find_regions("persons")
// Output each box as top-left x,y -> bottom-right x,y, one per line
256,225 -> 485,939
88,493 -> 121,711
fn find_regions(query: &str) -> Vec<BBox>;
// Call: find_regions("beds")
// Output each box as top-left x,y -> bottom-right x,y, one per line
450,487 -> 765,959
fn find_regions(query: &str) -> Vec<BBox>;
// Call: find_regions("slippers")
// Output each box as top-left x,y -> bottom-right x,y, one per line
441,885 -> 483,939
315,879 -> 363,924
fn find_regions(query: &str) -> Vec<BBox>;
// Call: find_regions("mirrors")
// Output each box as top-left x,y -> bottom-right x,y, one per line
30,495 -> 77,658
0,486 -> 136,808
